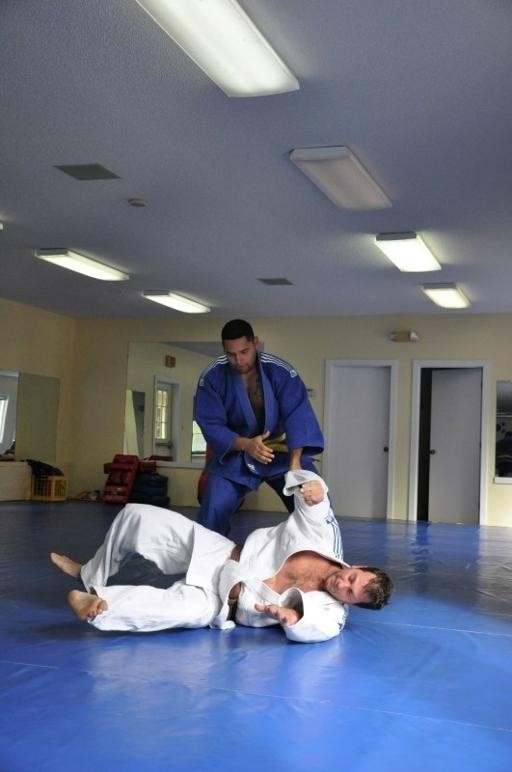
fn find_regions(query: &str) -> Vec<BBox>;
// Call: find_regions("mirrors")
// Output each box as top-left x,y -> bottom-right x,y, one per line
0,369 -> 19,463
121,342 -> 263,469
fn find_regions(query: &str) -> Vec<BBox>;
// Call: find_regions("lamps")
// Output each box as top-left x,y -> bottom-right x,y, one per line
35,249 -> 130,283
290,147 -> 391,210
372,232 -> 442,274
138,0 -> 300,97
142,290 -> 210,314
422,282 -> 470,310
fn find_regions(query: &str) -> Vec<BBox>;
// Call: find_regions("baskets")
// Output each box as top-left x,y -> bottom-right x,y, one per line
31,475 -> 68,501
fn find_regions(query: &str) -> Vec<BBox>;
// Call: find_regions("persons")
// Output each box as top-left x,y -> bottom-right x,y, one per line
50,467 -> 394,649
190,317 -> 333,537
